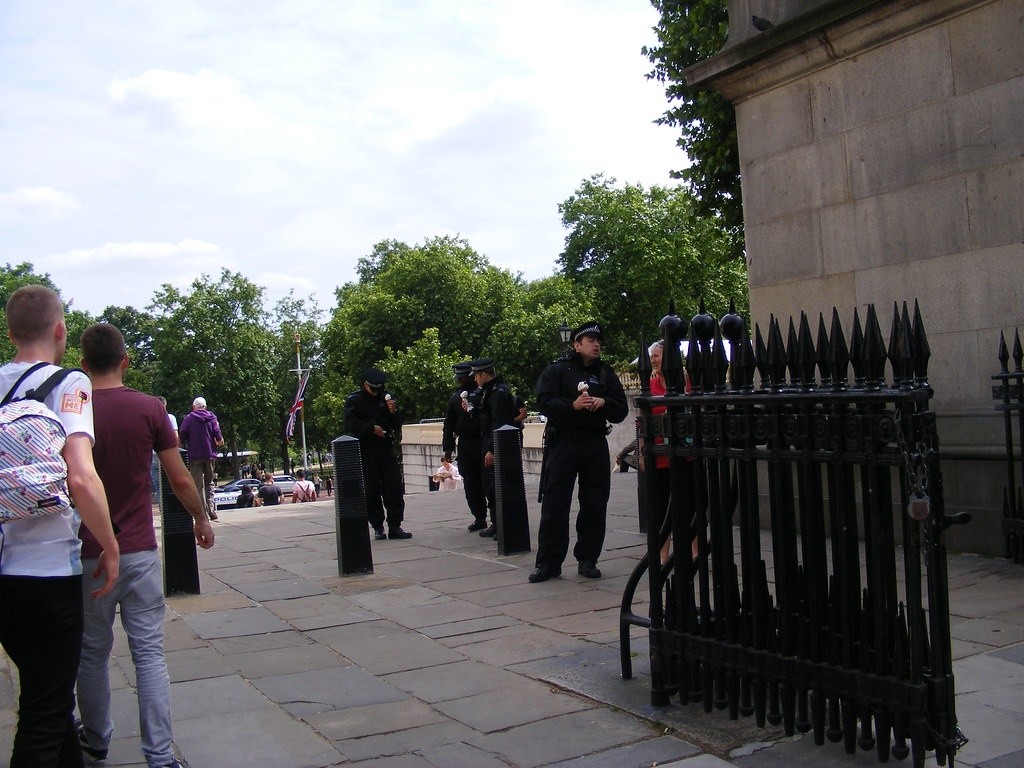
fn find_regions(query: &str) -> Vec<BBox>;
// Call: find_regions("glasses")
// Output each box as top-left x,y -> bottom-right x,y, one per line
658,339 -> 665,345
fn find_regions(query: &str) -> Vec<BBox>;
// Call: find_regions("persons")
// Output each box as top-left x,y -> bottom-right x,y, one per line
241,462 -> 266,479
290,459 -> 295,475
291,470 -> 319,504
0,285 -> 119,768
306,451 -> 334,468
75,323 -> 214,768
343,368 -> 412,540
442,357 -> 528,540
432,456 -> 462,492
640,340 -> 697,568
529,320 -> 628,583
313,472 -> 332,497
150,396 -> 223,521
235,474 -> 285,509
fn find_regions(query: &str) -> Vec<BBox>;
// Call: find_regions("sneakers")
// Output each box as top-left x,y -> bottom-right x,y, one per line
149,757 -> 187,768
73,718 -> 110,763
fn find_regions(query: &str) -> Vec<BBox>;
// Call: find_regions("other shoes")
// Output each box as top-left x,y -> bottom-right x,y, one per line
528,563 -> 563,583
387,525 -> 413,540
373,526 -> 387,540
478,522 -> 498,542
467,518 -> 488,531
577,559 -> 602,579
208,509 -> 217,520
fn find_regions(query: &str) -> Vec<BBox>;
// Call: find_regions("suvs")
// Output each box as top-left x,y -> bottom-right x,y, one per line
261,475 -> 315,495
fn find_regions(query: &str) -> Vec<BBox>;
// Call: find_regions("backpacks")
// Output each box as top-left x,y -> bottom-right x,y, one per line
296,482 -> 312,502
0,359 -> 91,569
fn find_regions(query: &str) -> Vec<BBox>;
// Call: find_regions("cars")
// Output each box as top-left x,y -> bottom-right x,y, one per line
219,478 -> 261,492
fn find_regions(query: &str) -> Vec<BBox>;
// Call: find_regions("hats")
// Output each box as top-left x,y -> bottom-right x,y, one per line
468,358 -> 496,377
571,321 -> 603,344
363,368 -> 388,395
451,362 -> 472,380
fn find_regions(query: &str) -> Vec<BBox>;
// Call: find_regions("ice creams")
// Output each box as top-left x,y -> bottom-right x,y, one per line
385,394 -> 391,402
460,391 -> 468,402
578,381 -> 590,394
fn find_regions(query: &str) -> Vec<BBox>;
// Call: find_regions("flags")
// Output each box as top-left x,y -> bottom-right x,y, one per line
286,369 -> 310,437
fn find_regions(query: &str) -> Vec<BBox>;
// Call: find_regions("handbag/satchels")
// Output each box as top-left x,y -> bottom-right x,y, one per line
251,493 -> 262,507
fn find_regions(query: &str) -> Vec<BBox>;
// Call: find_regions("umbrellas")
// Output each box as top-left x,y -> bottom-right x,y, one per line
631,338 -> 753,364
